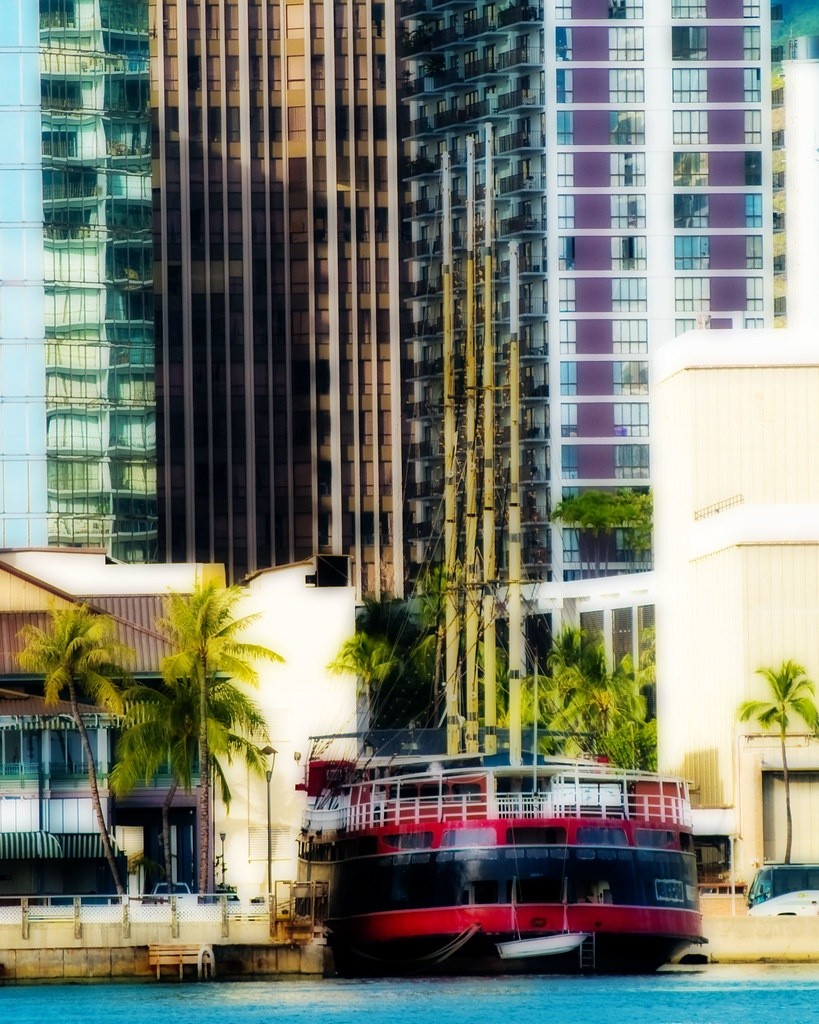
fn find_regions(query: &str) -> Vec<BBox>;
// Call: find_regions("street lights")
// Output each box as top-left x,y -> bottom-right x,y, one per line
220,831 -> 225,884
259,746 -> 279,894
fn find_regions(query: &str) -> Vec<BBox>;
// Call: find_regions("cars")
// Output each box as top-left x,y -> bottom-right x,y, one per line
216,888 -> 241,905
151,882 -> 190,895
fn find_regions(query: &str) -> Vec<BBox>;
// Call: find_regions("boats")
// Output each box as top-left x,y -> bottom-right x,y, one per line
295,155 -> 709,973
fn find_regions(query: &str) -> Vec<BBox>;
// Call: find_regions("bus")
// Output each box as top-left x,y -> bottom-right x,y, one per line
742,862 -> 819,917
